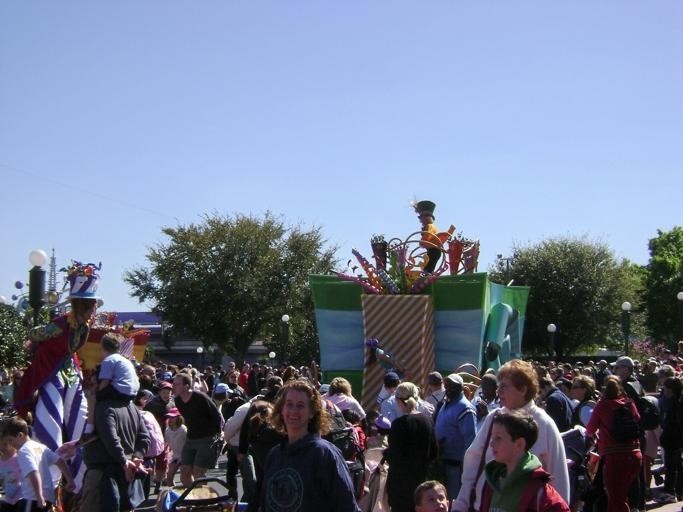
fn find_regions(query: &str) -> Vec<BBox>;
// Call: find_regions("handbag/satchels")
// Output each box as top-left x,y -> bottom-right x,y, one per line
609,405 -> 638,444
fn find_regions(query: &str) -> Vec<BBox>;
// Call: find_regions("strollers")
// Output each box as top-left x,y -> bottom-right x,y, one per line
560,425 -> 607,511
155,476 -> 234,512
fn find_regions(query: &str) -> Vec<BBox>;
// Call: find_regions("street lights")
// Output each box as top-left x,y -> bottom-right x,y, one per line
197,347 -> 203,369
29,250 -> 47,327
497,253 -> 517,284
622,301 -> 631,356
269,352 -> 275,367
678,292 -> 683,356
282,315 -> 289,343
547,324 -> 556,348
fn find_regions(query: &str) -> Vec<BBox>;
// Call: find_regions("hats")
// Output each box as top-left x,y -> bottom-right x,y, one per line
416,201 -> 436,221
609,356 -> 634,367
444,373 -> 463,385
215,383 -> 234,394
384,372 -> 399,384
164,407 -> 181,417
160,382 -> 172,389
596,360 -> 607,365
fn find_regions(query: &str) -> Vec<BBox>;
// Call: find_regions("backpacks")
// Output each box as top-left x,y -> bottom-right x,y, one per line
600,404 -> 640,443
323,399 -> 367,502
627,381 -> 660,431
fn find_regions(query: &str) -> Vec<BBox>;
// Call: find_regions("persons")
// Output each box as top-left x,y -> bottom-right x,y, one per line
537,376 -> 586,512
609,356 -> 647,512
451,358 -> 570,512
570,375 -> 608,512
3,416 -> 77,512
75,332 -> 140,448
173,373 -> 225,485
16,260 -> 104,452
135,360 -> 192,388
413,479 -> 450,512
479,410 -> 571,512
653,378 -> 683,504
242,379 -> 362,512
381,381 -> 438,512
79,364 -> 153,512
362,336 -> 406,415
529,356 -> 611,409
163,407 -> 188,486
238,376 -> 284,502
192,360 -> 323,404
374,372 -> 421,434
322,375 -> 367,424
586,375 -> 643,512
434,373 -> 478,502
633,345 -> 683,411
134,388 -> 156,504
223,390 -> 268,500
471,373 -> 503,431
425,367 -> 447,410
143,381 -> 177,495
0,420 -> 24,512
